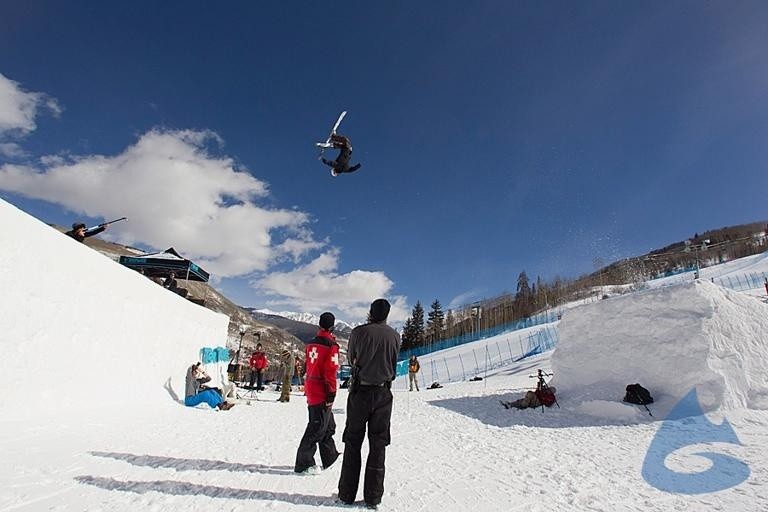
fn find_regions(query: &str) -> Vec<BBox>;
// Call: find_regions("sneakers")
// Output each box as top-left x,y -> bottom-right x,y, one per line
294,452 -> 343,475
338,492 -> 379,511
218,401 -> 235,410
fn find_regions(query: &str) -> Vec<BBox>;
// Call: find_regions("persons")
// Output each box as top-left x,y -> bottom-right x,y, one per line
61,221 -> 110,244
137,264 -> 178,293
320,135 -> 363,178
498,385 -> 558,409
181,363 -> 236,411
276,350 -> 296,403
292,311 -> 344,476
409,352 -> 420,391
333,300 -> 402,510
248,342 -> 268,392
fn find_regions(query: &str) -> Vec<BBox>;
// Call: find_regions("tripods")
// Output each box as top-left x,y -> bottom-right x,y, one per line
533,378 -> 560,413
242,363 -> 259,405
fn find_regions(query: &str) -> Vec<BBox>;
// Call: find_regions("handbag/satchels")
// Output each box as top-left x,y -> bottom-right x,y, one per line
623,383 -> 653,405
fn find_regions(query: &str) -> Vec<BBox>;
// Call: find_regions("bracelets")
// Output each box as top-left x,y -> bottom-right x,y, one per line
202,372 -> 205,375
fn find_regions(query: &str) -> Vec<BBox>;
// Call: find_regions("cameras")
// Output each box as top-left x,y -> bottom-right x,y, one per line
280,361 -> 286,367
196,362 -> 201,367
537,369 -> 542,373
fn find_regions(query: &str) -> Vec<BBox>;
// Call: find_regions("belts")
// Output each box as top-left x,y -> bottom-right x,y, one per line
360,381 -> 385,387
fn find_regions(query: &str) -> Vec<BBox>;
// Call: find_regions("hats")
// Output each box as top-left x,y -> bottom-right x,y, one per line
370,299 -> 390,321
319,312 -> 334,329
